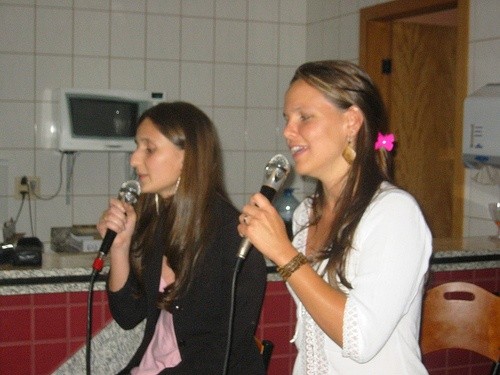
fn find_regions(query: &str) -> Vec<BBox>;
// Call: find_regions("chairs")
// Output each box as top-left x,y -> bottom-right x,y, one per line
418,281 -> 500,375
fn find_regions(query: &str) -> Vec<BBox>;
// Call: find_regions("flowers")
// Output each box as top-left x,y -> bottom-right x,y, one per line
373,132 -> 395,152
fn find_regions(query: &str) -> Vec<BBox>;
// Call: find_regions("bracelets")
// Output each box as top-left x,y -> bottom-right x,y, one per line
276,252 -> 308,281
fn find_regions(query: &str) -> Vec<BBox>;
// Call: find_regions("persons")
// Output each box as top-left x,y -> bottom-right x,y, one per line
97,101 -> 265,375
238,60 -> 432,375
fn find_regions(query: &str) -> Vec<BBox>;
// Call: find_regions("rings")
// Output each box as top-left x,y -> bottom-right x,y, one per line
243,214 -> 250,224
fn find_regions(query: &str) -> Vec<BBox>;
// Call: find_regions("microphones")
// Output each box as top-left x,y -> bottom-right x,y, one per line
92,180 -> 141,271
234,153 -> 290,259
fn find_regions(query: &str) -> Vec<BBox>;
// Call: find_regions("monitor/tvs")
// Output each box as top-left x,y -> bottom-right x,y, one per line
59,88 -> 164,153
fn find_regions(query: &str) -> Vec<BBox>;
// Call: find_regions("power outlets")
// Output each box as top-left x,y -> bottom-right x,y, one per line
15,175 -> 41,200
